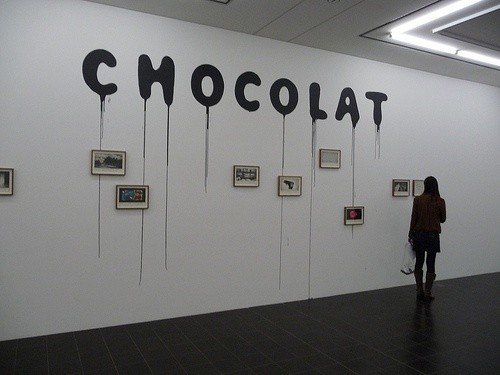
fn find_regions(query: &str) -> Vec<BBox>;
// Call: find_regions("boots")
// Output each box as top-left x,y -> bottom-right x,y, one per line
414,270 -> 425,298
425,272 -> 436,295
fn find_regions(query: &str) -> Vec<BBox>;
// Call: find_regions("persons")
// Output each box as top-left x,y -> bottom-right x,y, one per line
408,176 -> 446,301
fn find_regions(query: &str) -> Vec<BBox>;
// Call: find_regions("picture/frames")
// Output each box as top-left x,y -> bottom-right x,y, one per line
115,184 -> 149,210
0,168 -> 14,196
343,205 -> 365,226
413,180 -> 424,197
278,176 -> 302,197
90,149 -> 127,176
392,179 -> 411,197
233,165 -> 260,187
319,149 -> 342,169
